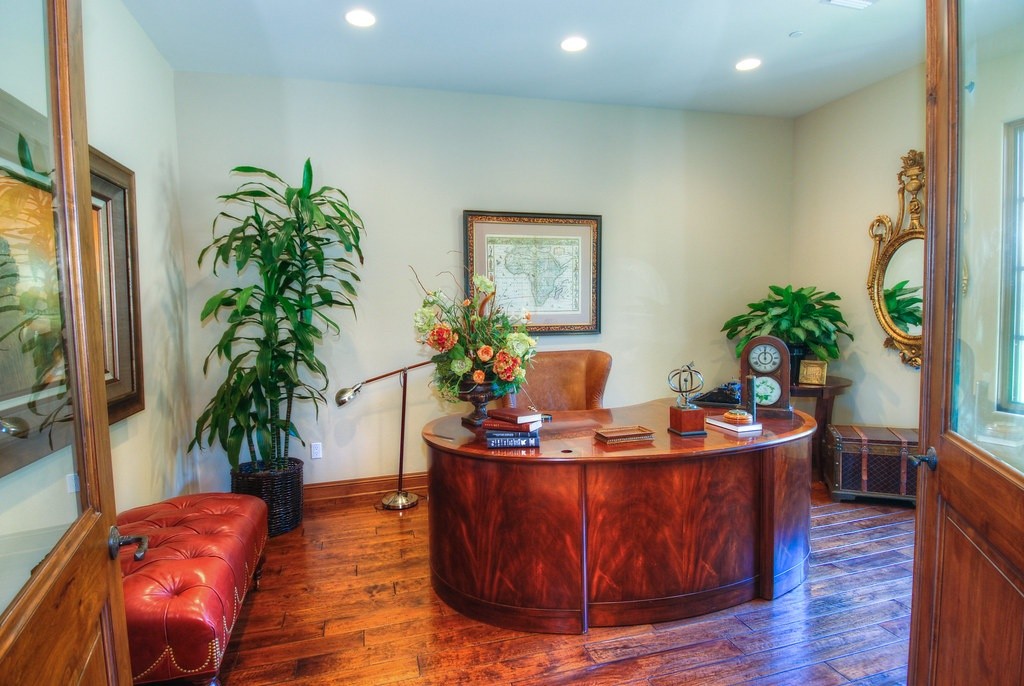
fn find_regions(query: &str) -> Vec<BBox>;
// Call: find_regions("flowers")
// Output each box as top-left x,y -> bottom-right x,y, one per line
408,250 -> 539,403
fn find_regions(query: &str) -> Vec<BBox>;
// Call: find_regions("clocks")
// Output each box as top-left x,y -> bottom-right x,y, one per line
735,336 -> 794,420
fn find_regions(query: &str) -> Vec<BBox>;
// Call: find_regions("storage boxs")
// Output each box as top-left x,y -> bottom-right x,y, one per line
819,424 -> 918,509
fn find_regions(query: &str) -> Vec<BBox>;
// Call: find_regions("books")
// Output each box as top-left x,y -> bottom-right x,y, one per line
706,415 -> 762,432
483,418 -> 542,432
486,431 -> 538,437
487,407 -> 541,424
487,436 -> 540,448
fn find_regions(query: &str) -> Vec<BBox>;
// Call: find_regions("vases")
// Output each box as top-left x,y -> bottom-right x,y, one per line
444,362 -> 501,427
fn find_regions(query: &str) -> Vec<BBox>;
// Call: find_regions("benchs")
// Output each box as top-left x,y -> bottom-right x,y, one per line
116,492 -> 268,686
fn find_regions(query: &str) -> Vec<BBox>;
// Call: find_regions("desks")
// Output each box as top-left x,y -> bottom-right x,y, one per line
790,376 -> 853,492
420,397 -> 818,637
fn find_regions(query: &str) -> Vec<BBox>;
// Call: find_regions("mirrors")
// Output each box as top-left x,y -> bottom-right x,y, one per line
866,149 -> 969,369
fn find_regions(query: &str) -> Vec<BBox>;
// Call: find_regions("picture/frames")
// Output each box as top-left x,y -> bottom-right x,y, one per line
0,88 -> 146,478
463,209 -> 601,337
799,360 -> 828,386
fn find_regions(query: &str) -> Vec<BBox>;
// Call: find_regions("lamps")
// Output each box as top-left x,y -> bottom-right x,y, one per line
336,359 -> 433,510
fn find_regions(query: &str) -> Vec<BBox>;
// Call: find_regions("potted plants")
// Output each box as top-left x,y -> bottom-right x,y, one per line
720,284 -> 854,386
187,156 -> 367,539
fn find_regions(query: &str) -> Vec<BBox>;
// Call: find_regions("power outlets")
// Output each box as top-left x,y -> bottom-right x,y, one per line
310,442 -> 322,459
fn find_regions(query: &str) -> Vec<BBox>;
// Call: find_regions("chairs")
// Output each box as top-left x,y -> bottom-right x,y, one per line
502,350 -> 613,410
